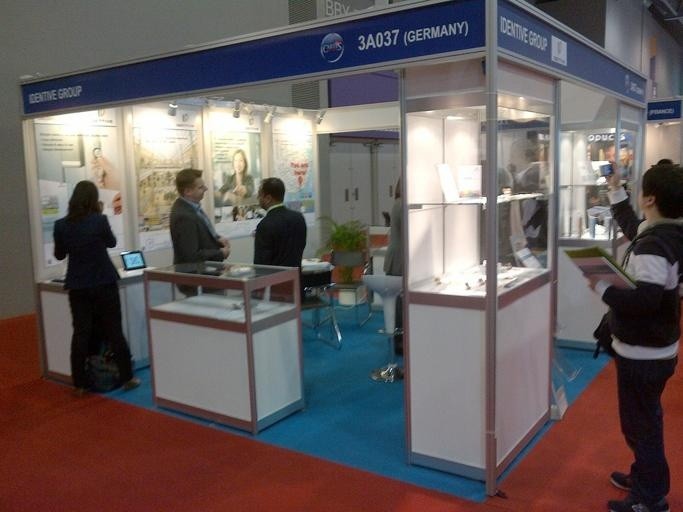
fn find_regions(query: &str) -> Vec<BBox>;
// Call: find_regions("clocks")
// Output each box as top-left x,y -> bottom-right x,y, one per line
119,249 -> 148,272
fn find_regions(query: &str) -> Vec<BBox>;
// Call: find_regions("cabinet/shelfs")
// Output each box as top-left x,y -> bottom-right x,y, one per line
143,260 -> 308,437
551,118 -> 649,358
38,262 -> 178,386
398,57 -> 561,483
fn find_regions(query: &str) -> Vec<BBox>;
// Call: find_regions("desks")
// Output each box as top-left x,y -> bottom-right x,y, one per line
299,257 -> 336,335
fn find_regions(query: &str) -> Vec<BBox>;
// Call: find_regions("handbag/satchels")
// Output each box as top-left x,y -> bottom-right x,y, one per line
589,311 -> 612,358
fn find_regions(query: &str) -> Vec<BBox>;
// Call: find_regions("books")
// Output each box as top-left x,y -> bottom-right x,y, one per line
564,245 -> 637,291
571,257 -> 636,291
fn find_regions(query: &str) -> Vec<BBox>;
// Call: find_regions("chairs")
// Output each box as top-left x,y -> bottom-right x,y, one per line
313,249 -> 375,330
299,270 -> 345,352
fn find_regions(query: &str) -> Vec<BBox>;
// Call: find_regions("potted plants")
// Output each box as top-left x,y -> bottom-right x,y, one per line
315,210 -> 371,257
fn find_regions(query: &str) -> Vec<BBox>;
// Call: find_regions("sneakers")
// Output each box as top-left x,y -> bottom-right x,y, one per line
611,471 -> 633,491
607,498 -> 670,511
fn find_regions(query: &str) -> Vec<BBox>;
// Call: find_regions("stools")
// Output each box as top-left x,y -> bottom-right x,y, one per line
360,273 -> 405,386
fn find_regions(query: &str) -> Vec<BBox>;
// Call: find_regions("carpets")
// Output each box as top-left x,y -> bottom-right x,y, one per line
98,292 -> 618,506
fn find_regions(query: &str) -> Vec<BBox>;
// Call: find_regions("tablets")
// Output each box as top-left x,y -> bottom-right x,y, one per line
120,250 -> 146,270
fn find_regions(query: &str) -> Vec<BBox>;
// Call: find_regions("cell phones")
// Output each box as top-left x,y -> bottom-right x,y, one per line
600,164 -> 612,177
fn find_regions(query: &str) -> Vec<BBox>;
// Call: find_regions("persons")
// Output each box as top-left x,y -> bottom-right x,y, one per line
214,150 -> 255,205
507,138 -> 549,248
52,180 -> 141,399
583,158 -> 683,511
380,176 -> 404,354
168,166 -> 231,299
251,176 -> 307,308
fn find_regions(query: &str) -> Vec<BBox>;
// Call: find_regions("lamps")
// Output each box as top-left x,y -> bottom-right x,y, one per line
168,96 -> 328,126
